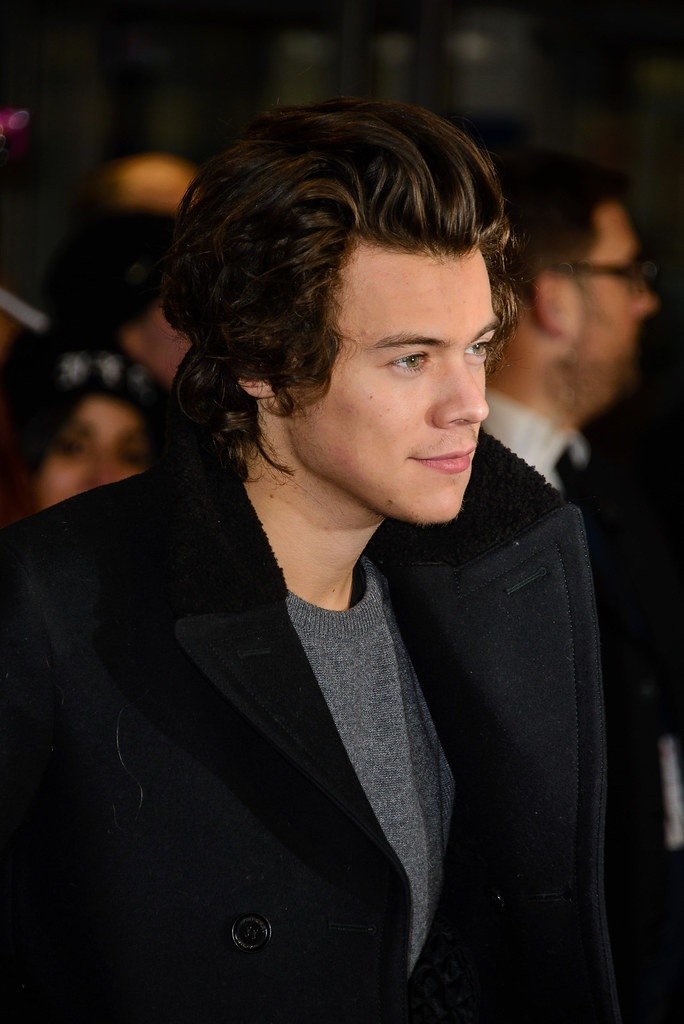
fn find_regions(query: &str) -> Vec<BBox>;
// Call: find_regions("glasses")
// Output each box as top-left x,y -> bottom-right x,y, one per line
547,249 -> 661,288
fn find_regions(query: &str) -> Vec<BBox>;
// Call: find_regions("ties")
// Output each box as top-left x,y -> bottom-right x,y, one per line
557,451 -> 589,501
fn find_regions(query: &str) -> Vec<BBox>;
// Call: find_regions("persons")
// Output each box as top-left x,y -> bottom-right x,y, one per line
0,123 -> 204,524
478,141 -> 684,1024
0,97 -> 620,1024
